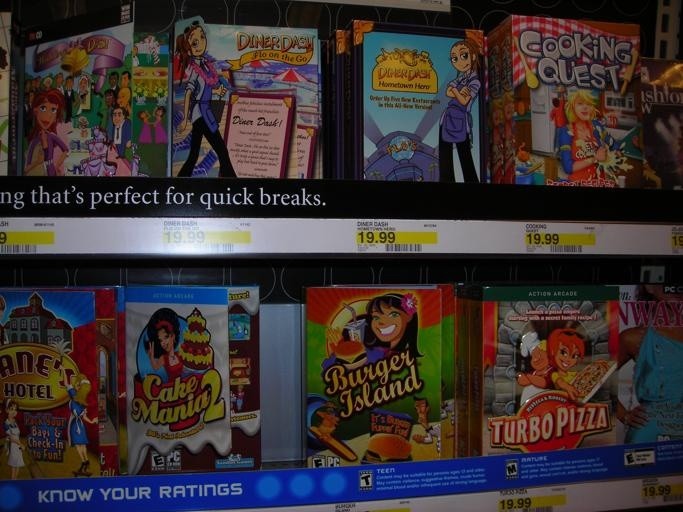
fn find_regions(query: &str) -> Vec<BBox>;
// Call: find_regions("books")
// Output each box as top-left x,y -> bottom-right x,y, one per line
1,15 -> 681,479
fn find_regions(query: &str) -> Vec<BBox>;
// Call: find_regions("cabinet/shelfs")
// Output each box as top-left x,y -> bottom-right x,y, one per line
1,176 -> 682,511
